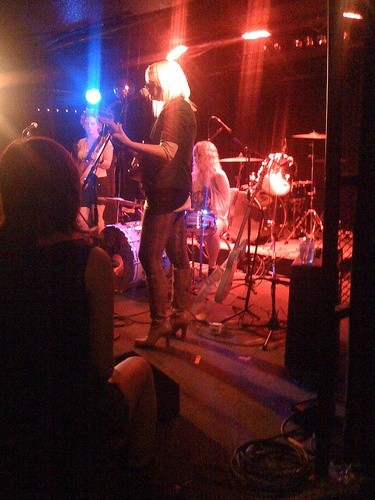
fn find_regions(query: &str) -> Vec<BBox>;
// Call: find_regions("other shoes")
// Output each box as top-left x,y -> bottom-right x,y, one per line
208,267 -> 215,277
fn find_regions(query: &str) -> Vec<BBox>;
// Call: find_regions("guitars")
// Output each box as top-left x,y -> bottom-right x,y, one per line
80,129 -> 111,190
95,109 -> 150,199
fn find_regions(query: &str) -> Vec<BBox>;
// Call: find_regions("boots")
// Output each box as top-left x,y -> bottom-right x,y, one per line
117,455 -> 180,500
135,269 -> 173,352
169,268 -> 193,341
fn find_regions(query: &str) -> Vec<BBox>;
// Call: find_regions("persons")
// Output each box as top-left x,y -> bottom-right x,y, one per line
111,60 -> 197,348
0,137 -> 159,500
192,141 -> 231,276
77,109 -> 114,231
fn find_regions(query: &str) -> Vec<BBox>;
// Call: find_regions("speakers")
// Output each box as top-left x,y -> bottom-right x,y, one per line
284,262 -> 335,372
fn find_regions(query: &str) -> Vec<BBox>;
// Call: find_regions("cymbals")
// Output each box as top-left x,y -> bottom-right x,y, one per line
219,157 -> 264,162
291,131 -> 326,140
96,197 -> 139,207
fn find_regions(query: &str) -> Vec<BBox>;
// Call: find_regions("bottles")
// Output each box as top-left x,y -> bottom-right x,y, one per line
298,242 -> 315,264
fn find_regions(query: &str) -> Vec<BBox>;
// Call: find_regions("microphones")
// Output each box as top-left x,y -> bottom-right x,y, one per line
212,116 -> 232,133
22,122 -> 38,134
125,87 -> 149,105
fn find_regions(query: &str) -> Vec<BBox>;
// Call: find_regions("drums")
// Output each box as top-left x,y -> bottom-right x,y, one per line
226,188 -> 286,245
258,153 -> 298,196
98,221 -> 171,294
286,181 -> 312,198
185,211 -> 217,237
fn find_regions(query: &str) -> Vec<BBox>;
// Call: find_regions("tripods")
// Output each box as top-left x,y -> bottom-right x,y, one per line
284,138 -> 323,244
217,147 -> 291,351
